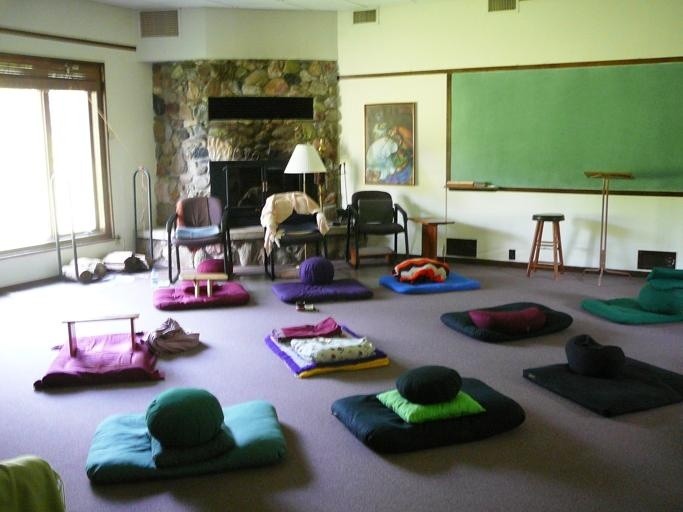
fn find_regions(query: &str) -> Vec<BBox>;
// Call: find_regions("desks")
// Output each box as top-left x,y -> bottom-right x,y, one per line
407,217 -> 456,263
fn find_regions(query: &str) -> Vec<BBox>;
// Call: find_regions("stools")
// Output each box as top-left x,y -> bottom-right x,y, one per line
182,273 -> 229,298
60,311 -> 141,359
526,213 -> 565,281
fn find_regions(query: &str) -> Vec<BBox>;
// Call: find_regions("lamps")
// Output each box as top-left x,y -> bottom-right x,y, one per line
282,143 -> 329,194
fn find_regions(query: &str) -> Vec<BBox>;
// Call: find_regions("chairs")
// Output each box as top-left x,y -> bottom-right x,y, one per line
164,190 -> 410,284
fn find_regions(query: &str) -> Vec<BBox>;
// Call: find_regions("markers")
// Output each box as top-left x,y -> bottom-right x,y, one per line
486,185 -> 498,188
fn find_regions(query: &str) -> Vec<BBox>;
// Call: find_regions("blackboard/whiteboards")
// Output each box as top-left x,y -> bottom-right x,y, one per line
446,58 -> 683,196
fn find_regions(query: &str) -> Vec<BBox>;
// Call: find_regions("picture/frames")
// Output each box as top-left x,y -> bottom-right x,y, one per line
362,102 -> 417,188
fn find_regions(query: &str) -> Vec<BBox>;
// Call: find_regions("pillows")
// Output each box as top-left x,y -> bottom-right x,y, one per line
149,421 -> 237,468
467,306 -> 547,334
645,265 -> 683,283
290,336 -> 377,365
375,386 -> 488,426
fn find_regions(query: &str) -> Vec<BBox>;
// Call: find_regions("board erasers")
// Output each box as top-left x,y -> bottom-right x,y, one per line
473,182 -> 489,188
